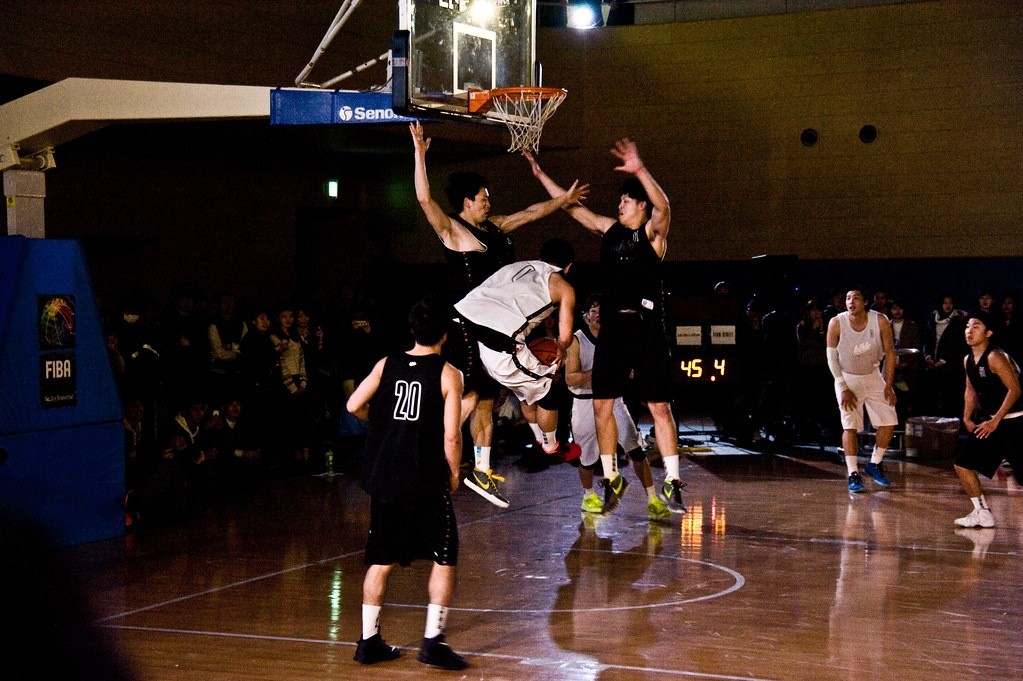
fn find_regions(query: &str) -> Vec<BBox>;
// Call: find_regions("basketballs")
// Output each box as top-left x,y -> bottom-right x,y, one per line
528,337 -> 558,367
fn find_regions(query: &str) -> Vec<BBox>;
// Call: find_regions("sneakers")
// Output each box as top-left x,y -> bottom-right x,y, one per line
531,439 -> 582,463
862,461 -> 891,486
463,469 -> 510,507
598,475 -> 630,516
353,624 -> 400,664
659,479 -> 687,514
580,492 -> 604,513
847,471 -> 866,493
954,509 -> 996,528
415,634 -> 468,671
648,500 -> 672,519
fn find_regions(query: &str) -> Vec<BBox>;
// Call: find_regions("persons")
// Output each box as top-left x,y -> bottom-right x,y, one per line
344,294 -> 468,670
409,120 -> 590,509
826,285 -> 898,493
744,287 -> 1023,445
564,296 -> 671,518
522,138 -> 691,515
457,238 -> 589,462
98,285 -> 380,523
945,311 -> 1023,527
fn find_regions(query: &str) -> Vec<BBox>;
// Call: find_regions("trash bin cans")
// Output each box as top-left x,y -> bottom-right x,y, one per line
904,417 -> 959,466
